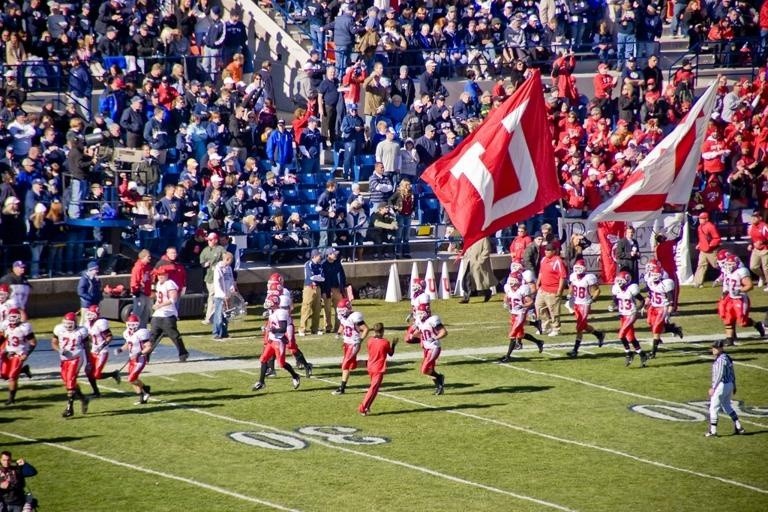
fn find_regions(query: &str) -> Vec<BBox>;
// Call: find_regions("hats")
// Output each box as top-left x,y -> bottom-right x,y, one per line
157,268 -> 168,275
82,3 -> 149,33
699,213 -> 709,220
204,233 -> 218,242
311,247 -> 339,259
88,262 -> 99,270
385,59 -> 446,135
278,49 -> 357,124
115,77 -> 208,103
751,210 -> 761,220
572,57 -> 657,178
713,341 -> 725,348
207,142 -> 261,195
468,2 -> 538,25
534,231 -> 556,251
5,147 -> 47,213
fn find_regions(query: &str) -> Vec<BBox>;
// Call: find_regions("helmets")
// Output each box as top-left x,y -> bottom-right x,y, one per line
59,305 -> 140,331
0,261 -> 25,324
572,260 -> 586,277
411,279 -> 425,292
263,273 -> 283,309
336,300 -> 352,317
508,262 -> 524,287
718,250 -> 739,273
646,259 -> 663,283
616,272 -> 632,287
418,304 -> 431,321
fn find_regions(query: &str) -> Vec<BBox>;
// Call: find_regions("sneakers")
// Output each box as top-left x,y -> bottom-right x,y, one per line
88,393 -> 101,398
134,386 -> 150,405
4,398 -> 15,405
332,388 -> 344,395
484,290 -> 492,302
23,363 -> 32,378
252,370 -> 276,391
179,353 -> 189,362
63,410 -> 74,417
598,331 -> 605,347
567,349 -> 577,357
755,321 -> 766,337
498,342 -> 522,363
291,361 -> 312,388
82,397 -> 88,414
535,319 -> 559,353
624,351 -> 655,368
114,371 -> 120,384
735,429 -> 745,434
459,299 -> 468,304
298,327 -> 337,337
705,431 -> 718,437
670,323 -> 684,338
435,375 -> 444,396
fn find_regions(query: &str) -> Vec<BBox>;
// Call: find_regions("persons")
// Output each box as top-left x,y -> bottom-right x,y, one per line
51,312 -> 92,418
84,304 -> 122,400
114,315 -> 152,405
703,339 -> 746,439
0,308 -> 38,405
359,322 -> 400,417
0,0 -> 768,397
0,450 -> 38,511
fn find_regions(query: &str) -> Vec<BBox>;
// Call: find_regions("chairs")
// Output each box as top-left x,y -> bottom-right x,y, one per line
280,169 -> 343,251
